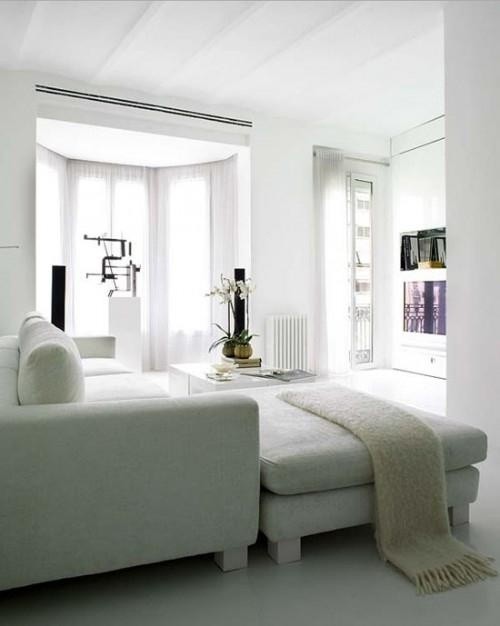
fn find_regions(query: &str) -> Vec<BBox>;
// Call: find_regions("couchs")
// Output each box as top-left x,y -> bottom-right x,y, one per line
0,312 -> 264,594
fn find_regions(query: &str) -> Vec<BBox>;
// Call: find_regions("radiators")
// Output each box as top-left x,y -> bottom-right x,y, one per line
267,313 -> 307,375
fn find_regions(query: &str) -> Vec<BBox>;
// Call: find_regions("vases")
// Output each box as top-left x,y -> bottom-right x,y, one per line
222,342 -> 253,358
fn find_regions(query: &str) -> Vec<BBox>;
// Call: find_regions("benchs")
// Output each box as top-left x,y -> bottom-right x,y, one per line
244,381 -> 489,564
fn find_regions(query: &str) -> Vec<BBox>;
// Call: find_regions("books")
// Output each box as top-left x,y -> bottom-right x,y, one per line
396,226 -> 446,271
239,366 -> 283,378
268,368 -> 320,383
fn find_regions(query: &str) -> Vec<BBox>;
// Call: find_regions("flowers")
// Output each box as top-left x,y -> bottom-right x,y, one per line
204,275 -> 259,350
